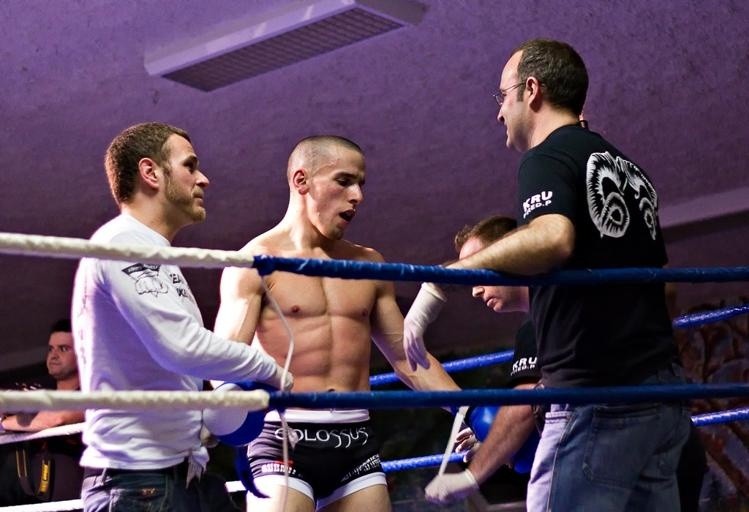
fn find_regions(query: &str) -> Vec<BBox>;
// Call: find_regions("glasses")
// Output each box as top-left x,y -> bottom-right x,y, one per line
492,79 -> 546,106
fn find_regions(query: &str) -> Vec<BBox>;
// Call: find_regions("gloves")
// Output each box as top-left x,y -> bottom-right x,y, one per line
403,283 -> 448,372
425,469 -> 482,509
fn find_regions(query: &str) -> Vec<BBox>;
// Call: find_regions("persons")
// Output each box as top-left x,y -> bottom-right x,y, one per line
424,215 -> 708,512
1,319 -> 88,501
71,122 -> 295,512
400,35 -> 694,510
208,134 -> 537,512
